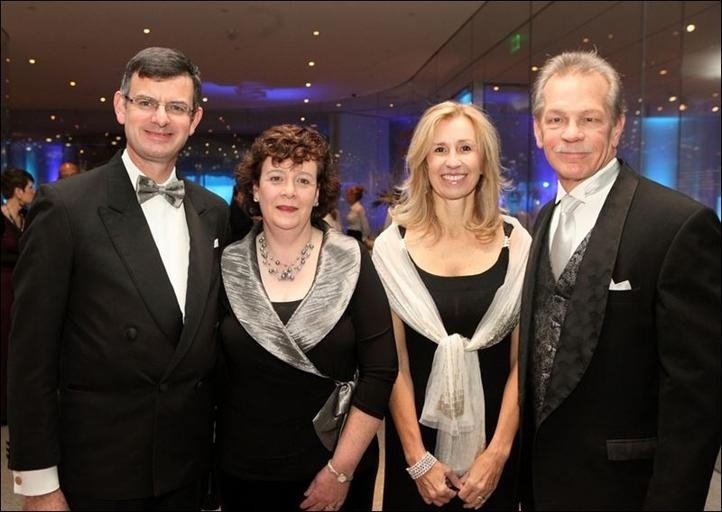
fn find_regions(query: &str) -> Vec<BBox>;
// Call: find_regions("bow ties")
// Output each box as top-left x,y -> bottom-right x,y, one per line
136,175 -> 186,208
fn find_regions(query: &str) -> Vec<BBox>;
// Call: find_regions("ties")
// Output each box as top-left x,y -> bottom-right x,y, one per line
550,160 -> 620,282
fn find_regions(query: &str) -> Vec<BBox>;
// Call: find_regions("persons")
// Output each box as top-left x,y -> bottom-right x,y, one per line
517,50 -> 721,511
57,162 -> 81,181
371,99 -> 534,512
228,182 -> 248,212
4,45 -> 255,512
0,168 -> 36,428
209,123 -> 398,511
345,185 -> 374,252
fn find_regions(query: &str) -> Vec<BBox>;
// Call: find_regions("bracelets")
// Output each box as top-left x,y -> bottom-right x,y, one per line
406,450 -> 438,480
327,458 -> 355,484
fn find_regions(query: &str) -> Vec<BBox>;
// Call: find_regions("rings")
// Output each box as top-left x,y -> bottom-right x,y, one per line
476,496 -> 485,503
326,504 -> 336,511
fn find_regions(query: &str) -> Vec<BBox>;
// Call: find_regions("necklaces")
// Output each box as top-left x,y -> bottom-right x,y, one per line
259,232 -> 314,280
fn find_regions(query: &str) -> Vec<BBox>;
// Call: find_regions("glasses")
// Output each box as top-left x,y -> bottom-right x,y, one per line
124,95 -> 196,114
